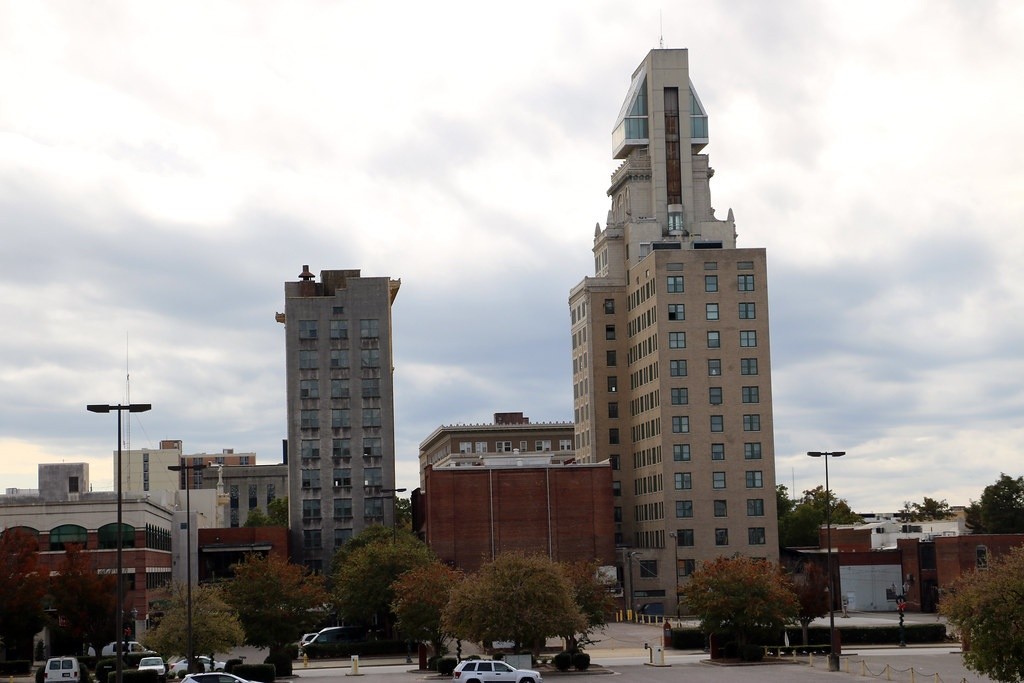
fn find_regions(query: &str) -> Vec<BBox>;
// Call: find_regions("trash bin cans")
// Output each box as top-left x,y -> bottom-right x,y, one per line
710,632 -> 719,659
419,644 -> 427,670
663,622 -> 672,648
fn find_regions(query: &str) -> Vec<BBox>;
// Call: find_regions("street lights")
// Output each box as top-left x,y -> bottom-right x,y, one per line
890,581 -> 910,647
381,488 -> 406,543
167,464 -> 208,672
808,451 -> 846,671
120,608 -> 138,667
87,403 -> 151,683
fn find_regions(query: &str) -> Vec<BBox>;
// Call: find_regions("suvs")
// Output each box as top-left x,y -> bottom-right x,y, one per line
453,658 -> 543,683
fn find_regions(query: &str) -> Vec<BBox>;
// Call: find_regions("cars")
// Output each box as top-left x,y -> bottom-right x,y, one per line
300,624 -> 368,656
44,639 -> 263,683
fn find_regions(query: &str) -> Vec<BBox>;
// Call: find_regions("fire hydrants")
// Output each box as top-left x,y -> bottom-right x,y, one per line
302,653 -> 309,668
